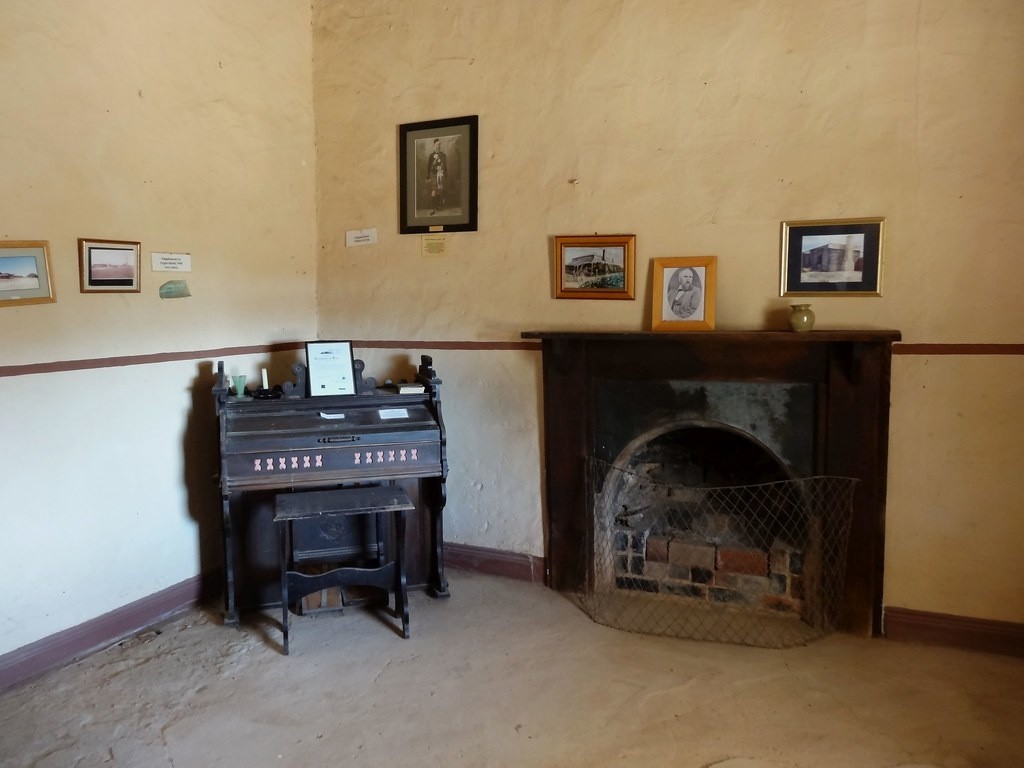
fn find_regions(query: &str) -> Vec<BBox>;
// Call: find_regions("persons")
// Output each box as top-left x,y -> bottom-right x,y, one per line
426,138 -> 448,215
668,269 -> 701,318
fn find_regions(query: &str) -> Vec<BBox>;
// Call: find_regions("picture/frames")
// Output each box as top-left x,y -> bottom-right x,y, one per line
400,115 -> 478,235
0,239 -> 58,307
779,217 -> 887,298
78,237 -> 142,293
651,256 -> 716,332
553,234 -> 636,301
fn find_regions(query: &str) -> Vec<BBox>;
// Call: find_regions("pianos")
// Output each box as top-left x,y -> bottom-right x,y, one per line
208,358 -> 452,627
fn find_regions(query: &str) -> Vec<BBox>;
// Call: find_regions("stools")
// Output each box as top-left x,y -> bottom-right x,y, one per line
273,484 -> 415,655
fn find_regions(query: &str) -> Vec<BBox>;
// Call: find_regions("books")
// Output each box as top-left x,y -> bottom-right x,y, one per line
398,383 -> 425,394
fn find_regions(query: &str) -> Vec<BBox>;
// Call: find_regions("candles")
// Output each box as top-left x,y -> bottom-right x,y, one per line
261,368 -> 269,389
